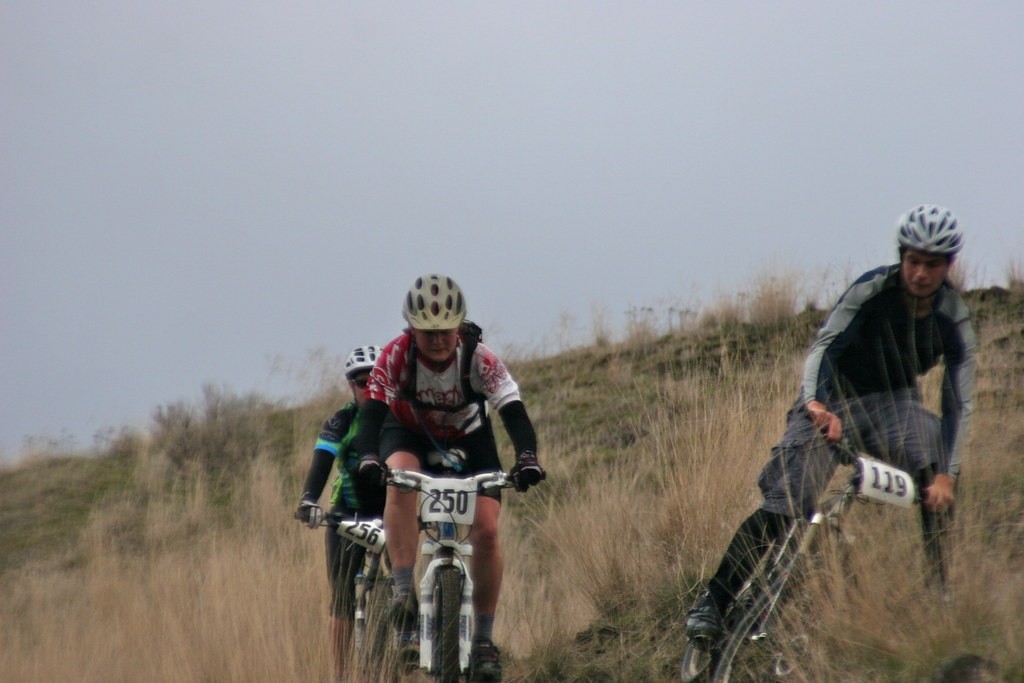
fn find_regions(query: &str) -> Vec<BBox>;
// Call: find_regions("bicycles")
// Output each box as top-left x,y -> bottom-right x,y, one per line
289,461 -> 547,683
679,408 -> 942,683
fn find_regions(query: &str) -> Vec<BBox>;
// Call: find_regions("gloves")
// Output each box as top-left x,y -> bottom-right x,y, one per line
504,450 -> 542,492
357,454 -> 389,486
297,499 -> 324,530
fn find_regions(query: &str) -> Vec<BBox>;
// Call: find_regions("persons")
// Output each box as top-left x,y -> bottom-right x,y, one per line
298,347 -> 420,683
685,203 -> 977,644
355,273 -> 545,683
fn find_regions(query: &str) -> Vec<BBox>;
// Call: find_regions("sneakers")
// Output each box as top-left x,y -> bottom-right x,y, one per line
468,642 -> 501,683
389,593 -> 418,633
684,587 -> 728,647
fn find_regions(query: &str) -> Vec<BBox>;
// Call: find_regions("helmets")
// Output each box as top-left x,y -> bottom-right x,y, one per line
401,273 -> 467,331
344,345 -> 384,375
893,202 -> 966,256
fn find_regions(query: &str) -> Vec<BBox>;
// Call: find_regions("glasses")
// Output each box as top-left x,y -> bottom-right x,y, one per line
350,376 -> 368,389
